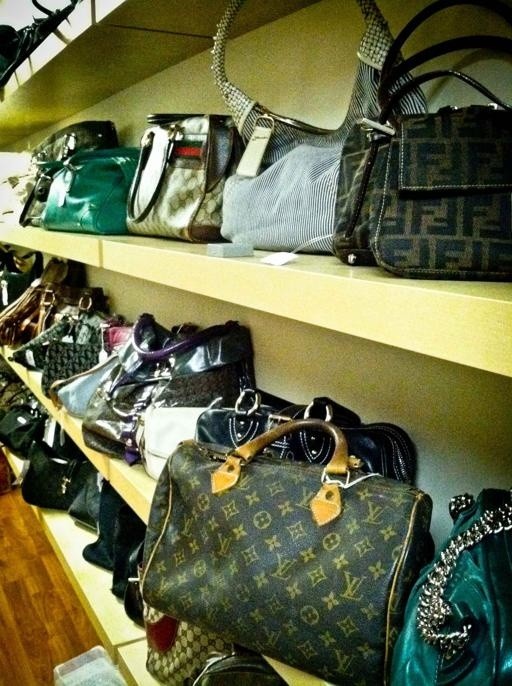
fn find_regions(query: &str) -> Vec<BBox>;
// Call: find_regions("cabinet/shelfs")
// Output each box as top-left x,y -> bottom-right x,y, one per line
1,0 -> 512,684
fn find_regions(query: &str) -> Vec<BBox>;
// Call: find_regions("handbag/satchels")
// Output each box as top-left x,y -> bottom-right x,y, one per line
210,0 -> 428,254
331,0 -> 512,280
121,388 -> 512,686
0,0 -> 83,88
6,120 -> 120,227
0,241 -> 254,596
41,147 -> 140,235
125,113 -> 247,243
